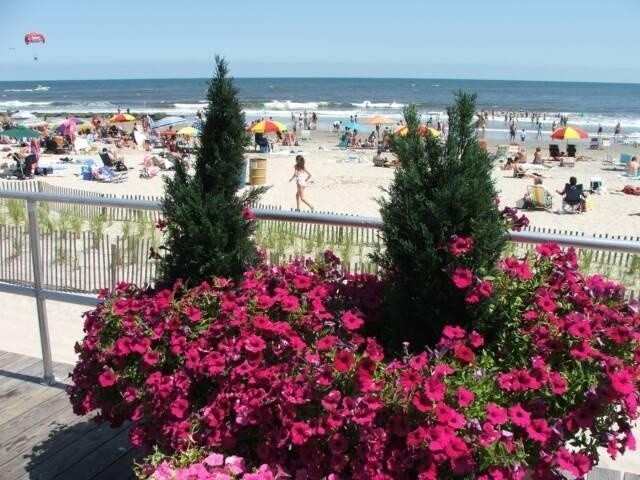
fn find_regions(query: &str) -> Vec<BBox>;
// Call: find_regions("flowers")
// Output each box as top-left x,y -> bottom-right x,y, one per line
64,240 -> 640,480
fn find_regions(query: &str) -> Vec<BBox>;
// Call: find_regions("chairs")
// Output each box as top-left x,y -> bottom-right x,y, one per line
254,132 -> 273,153
549,143 -> 576,161
526,184 -> 585,215
97,152 -> 127,171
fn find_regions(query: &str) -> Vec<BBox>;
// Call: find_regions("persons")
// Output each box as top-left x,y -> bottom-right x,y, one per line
289,155 -> 314,212
333,114 -> 397,170
473,108 -> 640,215
1,102 -> 208,180
248,108 -> 318,152
426,116 -> 445,137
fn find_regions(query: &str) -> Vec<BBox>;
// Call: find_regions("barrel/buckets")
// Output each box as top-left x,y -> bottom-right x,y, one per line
250,158 -> 267,185
238,165 -> 246,189
